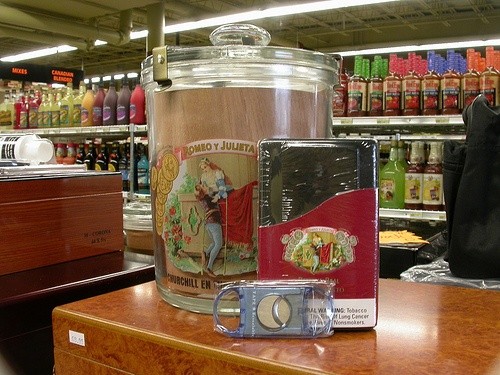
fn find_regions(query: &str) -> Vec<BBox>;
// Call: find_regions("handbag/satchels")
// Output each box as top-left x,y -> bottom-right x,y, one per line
441,93 -> 500,278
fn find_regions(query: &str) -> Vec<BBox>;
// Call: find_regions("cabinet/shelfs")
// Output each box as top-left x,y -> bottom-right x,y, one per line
0,123 -> 153,201
330,114 -> 467,224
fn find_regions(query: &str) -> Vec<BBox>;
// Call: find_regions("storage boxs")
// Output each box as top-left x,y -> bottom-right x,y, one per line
0,170 -> 125,277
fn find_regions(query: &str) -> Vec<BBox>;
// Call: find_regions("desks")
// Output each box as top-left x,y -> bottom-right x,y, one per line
51,272 -> 500,375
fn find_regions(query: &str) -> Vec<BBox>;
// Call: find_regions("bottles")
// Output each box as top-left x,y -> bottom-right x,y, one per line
0,77 -> 146,130
376,140 -> 449,212
329,45 -> 500,116
0,132 -> 150,194
138,23 -> 340,316
